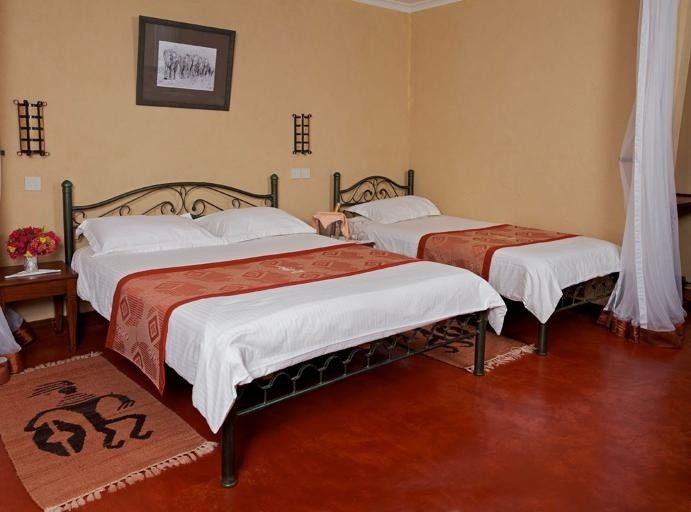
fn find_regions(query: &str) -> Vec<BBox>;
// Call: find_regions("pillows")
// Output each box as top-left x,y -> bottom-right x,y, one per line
342,194 -> 443,224
77,210 -> 222,256
198,201 -> 318,244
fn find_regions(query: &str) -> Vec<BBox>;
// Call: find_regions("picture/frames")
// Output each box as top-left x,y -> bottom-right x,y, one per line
136,15 -> 237,111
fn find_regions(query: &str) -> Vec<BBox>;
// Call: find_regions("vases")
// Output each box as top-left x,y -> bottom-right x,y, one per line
25,257 -> 38,273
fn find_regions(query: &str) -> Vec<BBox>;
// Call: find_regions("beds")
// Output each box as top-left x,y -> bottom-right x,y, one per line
330,172 -> 626,356
60,174 -> 508,487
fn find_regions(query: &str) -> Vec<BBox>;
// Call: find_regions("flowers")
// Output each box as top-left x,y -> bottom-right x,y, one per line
5,228 -> 62,255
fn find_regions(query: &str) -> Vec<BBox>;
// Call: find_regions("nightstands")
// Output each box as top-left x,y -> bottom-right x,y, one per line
0,260 -> 80,351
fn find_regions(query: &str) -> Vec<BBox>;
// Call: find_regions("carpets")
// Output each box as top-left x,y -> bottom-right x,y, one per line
402,320 -> 535,374
0,350 -> 215,512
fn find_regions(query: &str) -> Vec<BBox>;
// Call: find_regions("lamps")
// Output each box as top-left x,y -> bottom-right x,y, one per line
14,98 -> 50,157
292,113 -> 313,155
